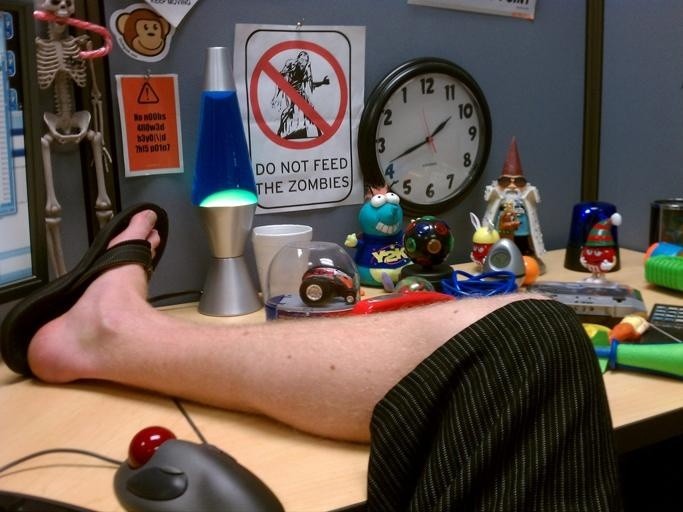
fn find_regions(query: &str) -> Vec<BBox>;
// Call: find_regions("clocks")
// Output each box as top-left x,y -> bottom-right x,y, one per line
355,54 -> 494,220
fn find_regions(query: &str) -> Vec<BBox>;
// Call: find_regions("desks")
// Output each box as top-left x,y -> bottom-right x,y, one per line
1,242 -> 683,511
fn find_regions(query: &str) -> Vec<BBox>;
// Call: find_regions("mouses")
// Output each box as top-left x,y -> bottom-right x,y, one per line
113,427 -> 285,512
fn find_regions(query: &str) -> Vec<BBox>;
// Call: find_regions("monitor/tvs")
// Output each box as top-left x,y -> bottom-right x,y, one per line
1,0 -> 49,305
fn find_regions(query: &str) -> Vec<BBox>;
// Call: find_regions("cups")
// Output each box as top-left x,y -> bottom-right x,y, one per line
252,223 -> 314,305
560,201 -> 620,272
649,197 -> 682,249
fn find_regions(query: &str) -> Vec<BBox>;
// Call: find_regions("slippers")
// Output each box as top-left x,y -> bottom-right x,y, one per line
1,199 -> 169,378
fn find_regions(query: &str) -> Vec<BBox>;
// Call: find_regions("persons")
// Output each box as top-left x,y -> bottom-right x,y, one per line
481,136 -> 547,267
1,201 -> 625,511
